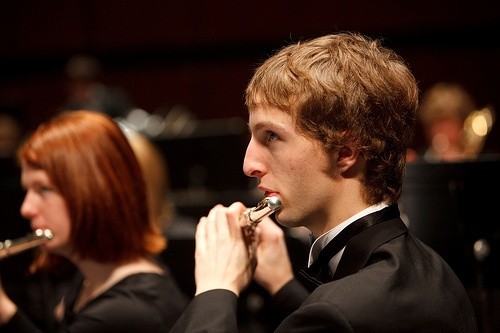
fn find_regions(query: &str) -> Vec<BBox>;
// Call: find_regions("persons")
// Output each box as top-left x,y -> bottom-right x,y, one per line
0,111 -> 189,333
419,85 -> 473,162
171,34 -> 477,333
0,115 -> 27,293
120,121 -> 170,255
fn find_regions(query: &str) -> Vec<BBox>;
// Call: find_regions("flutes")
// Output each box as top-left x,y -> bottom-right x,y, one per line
0,227 -> 53,258
238,194 -> 282,231
